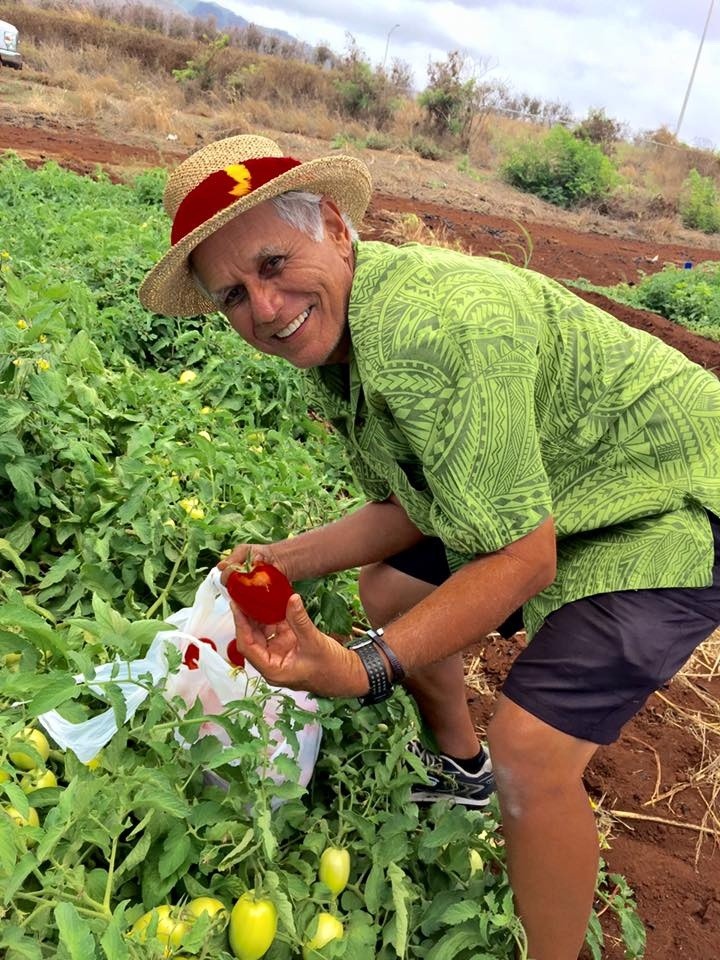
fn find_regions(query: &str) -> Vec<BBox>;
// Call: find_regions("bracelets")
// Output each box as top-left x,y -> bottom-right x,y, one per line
366,628 -> 405,683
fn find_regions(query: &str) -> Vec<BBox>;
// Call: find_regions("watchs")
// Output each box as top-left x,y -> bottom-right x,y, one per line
346,635 -> 394,707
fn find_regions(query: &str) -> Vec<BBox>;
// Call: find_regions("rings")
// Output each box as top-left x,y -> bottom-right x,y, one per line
266,634 -> 276,642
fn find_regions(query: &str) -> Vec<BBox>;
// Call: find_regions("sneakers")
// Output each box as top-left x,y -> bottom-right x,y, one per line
368,738 -> 495,806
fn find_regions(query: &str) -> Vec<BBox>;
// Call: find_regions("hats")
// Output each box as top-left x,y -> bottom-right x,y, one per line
139,134 -> 372,318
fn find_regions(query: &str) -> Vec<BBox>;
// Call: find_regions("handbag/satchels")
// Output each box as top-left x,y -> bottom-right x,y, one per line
37,567 -> 323,816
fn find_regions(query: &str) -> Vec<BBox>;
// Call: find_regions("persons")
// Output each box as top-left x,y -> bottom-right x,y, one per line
137,133 -> 719,960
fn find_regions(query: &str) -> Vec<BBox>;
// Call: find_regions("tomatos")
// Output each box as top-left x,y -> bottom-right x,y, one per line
0,368 -> 354,960
